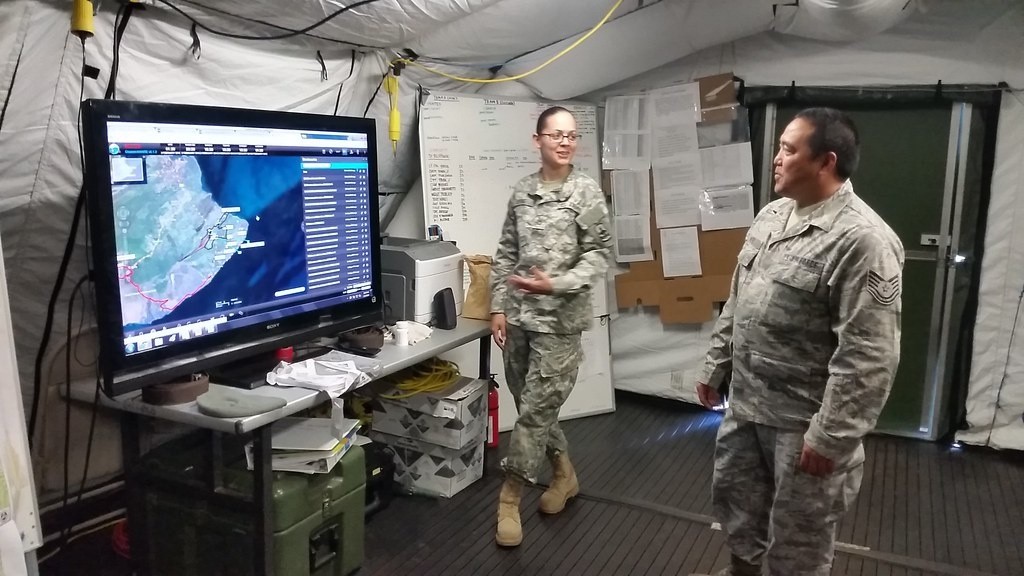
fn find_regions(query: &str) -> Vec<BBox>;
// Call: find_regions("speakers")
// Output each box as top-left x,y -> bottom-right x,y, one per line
435,287 -> 457,330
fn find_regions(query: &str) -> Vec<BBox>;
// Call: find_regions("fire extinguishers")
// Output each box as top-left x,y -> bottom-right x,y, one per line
478,372 -> 499,448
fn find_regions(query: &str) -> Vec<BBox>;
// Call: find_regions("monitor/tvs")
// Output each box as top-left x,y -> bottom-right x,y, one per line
81,98 -> 383,398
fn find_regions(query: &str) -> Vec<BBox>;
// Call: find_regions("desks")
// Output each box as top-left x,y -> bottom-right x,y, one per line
58,314 -> 493,576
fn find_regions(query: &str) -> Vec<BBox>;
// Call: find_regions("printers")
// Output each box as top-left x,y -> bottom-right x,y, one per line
379,232 -> 464,325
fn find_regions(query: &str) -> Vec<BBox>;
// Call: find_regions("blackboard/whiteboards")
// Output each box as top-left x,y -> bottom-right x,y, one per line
414,86 -> 618,435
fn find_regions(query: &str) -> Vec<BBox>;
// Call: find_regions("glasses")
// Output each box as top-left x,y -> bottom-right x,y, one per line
538,130 -> 583,143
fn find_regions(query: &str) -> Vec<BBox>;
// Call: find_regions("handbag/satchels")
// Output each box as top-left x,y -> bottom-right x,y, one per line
460,254 -> 493,322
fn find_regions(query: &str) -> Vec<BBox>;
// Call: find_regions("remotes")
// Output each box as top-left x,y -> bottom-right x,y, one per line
333,342 -> 381,359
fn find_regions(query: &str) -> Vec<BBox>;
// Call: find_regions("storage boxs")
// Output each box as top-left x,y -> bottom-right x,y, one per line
367,372 -> 490,450
129,427 -> 366,576
363,431 -> 486,499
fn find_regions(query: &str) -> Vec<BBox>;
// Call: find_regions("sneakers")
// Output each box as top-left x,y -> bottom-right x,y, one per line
540,464 -> 580,513
495,478 -> 524,546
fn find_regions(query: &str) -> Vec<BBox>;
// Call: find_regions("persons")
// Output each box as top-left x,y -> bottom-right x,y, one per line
697,108 -> 906,576
488,107 -> 613,546
